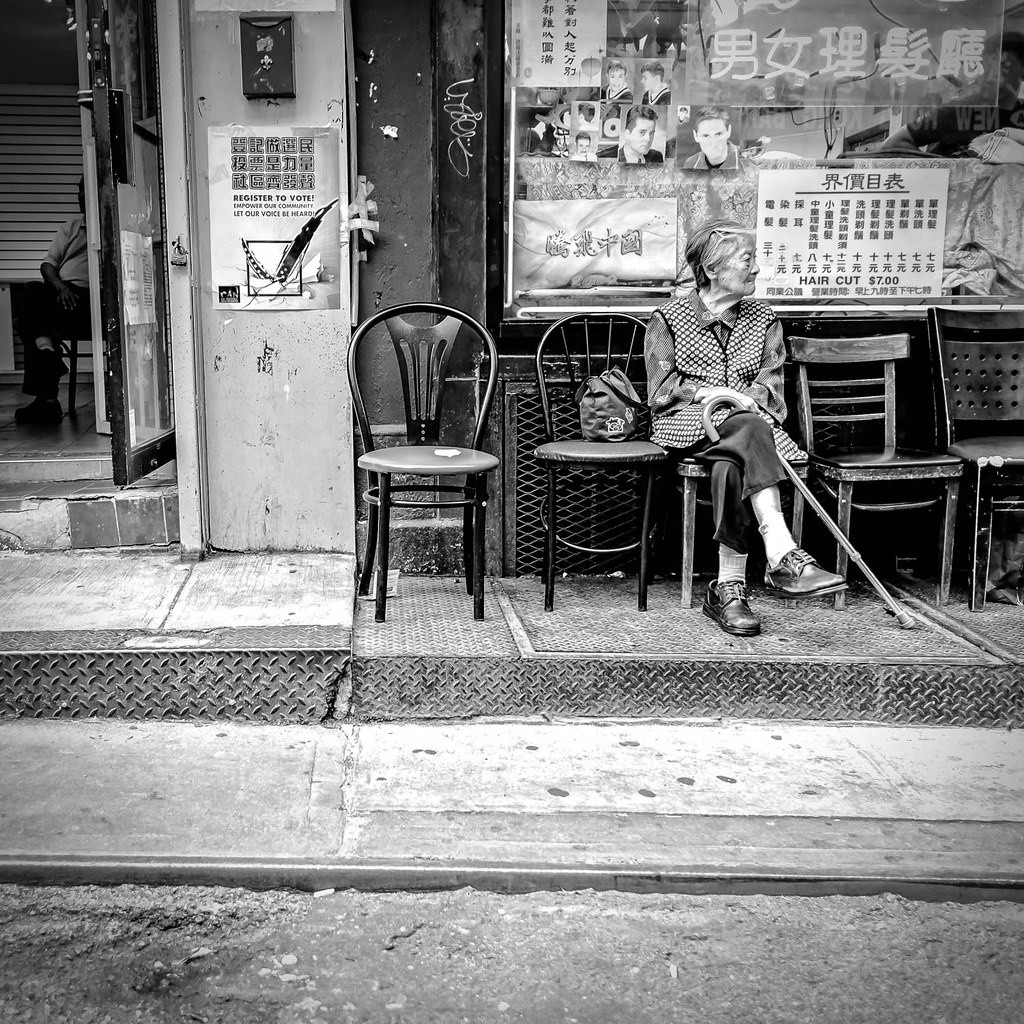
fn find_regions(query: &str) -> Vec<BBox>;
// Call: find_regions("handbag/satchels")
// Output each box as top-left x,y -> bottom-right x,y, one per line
574,366 -> 650,443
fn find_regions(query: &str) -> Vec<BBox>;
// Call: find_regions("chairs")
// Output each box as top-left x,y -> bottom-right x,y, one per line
538,304 -> 1024,610
345,301 -> 499,622
57,326 -> 108,414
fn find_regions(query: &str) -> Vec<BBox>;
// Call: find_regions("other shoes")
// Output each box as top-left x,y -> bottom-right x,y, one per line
14,398 -> 63,424
28,348 -> 70,381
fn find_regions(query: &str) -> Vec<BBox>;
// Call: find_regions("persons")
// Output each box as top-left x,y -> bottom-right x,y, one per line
643,220 -> 849,635
572,60 -> 739,171
15,176 -> 105,426
879,31 -> 1024,154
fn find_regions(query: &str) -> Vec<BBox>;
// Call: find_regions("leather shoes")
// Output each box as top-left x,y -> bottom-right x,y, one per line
764,548 -> 849,601
703,579 -> 762,637
